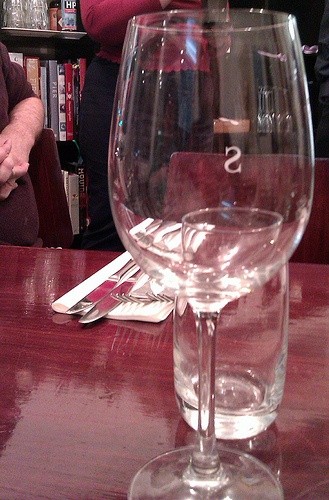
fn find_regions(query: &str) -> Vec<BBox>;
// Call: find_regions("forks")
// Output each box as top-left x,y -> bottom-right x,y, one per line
114,274 -> 177,305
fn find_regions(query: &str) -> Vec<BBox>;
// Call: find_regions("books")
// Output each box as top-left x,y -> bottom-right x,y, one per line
8,52 -> 87,141
0,0 -> 78,31
61,165 -> 90,235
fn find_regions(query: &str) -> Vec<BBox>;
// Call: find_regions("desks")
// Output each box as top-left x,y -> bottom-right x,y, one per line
0,245 -> 329,500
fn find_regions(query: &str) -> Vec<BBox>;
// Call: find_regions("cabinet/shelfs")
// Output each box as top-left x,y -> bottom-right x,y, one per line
0,0 -> 117,250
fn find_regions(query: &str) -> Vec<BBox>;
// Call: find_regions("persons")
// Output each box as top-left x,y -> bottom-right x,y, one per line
76,0 -> 211,250
0,42 -> 45,248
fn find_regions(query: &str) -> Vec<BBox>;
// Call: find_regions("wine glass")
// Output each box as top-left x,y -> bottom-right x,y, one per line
106,8 -> 313,500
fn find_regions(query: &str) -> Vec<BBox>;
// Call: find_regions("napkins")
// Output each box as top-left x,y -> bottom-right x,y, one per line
52,250 -> 175,323
128,216 -> 216,264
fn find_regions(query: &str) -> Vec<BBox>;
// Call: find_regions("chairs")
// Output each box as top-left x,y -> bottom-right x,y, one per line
10,128 -> 74,249
163,153 -> 329,265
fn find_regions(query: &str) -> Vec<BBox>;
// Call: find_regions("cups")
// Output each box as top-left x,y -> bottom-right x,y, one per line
171,207 -> 283,262
173,262 -> 289,440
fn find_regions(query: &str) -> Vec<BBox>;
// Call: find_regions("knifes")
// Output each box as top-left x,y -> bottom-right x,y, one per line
66,259 -> 143,333
